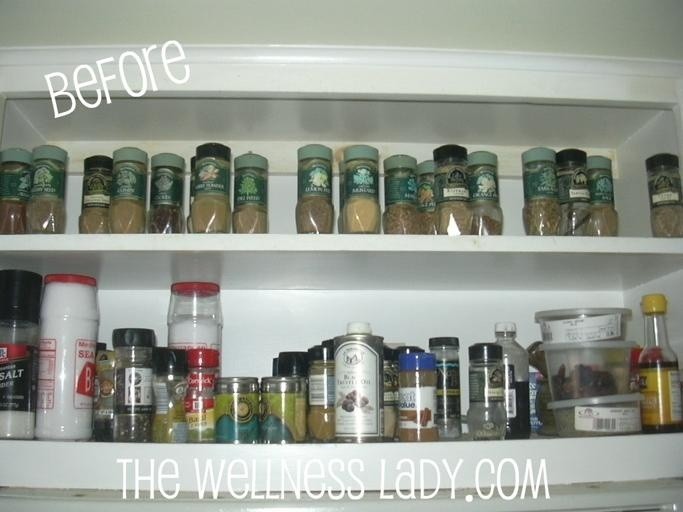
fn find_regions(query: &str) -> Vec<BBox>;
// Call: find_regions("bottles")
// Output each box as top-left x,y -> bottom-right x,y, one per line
25,144 -> 68,235
297,142 -> 503,233
521,146 -> 561,237
0,268 -> 681,443
555,146 -> 590,234
76,142 -> 268,237
1,147 -> 31,233
643,154 -> 681,237
585,156 -> 620,234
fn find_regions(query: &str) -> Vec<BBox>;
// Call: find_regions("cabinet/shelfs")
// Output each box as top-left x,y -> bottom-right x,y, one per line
1,38 -> 681,510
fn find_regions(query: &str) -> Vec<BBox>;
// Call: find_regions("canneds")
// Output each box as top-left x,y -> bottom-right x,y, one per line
214,377 -> 262,444
260,377 -> 307,444
